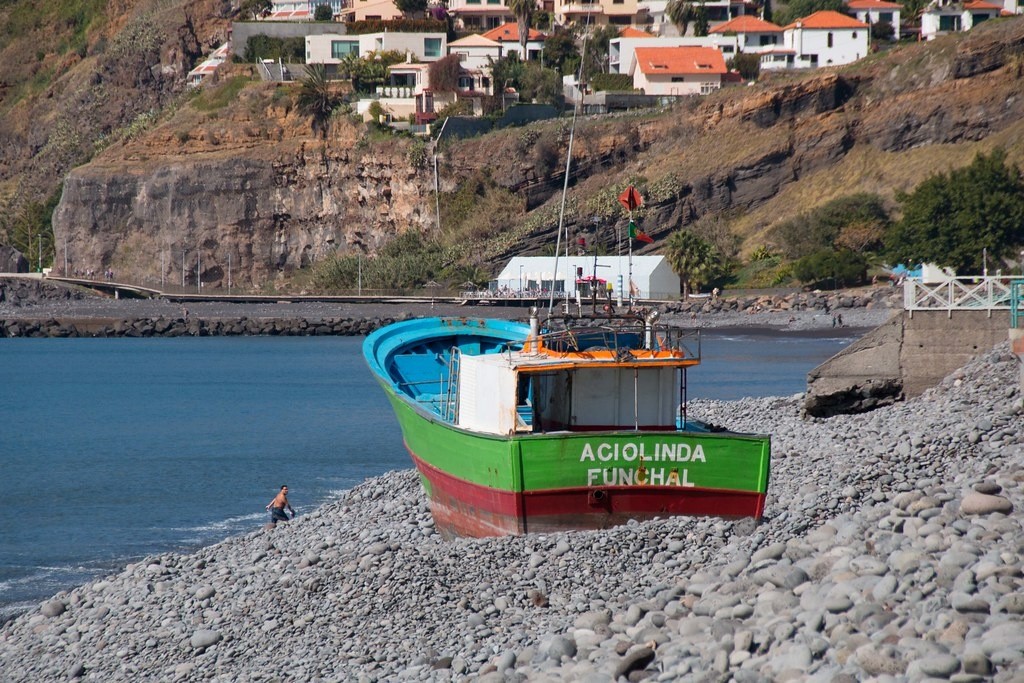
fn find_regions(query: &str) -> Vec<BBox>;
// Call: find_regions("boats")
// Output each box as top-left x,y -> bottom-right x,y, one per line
360,1 -> 772,542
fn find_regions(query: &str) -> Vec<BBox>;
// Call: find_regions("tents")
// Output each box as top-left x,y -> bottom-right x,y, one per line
497,255 -> 680,302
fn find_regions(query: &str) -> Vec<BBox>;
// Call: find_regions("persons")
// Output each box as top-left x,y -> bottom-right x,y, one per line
266,486 -> 296,523
872,276 -> 878,288
832,314 -> 842,328
691,312 -> 697,323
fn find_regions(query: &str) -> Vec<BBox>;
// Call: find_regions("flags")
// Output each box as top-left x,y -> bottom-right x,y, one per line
629,222 -> 654,243
630,281 -> 639,297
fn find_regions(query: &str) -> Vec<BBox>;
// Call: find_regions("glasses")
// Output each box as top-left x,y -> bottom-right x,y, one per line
282,489 -> 288,490
